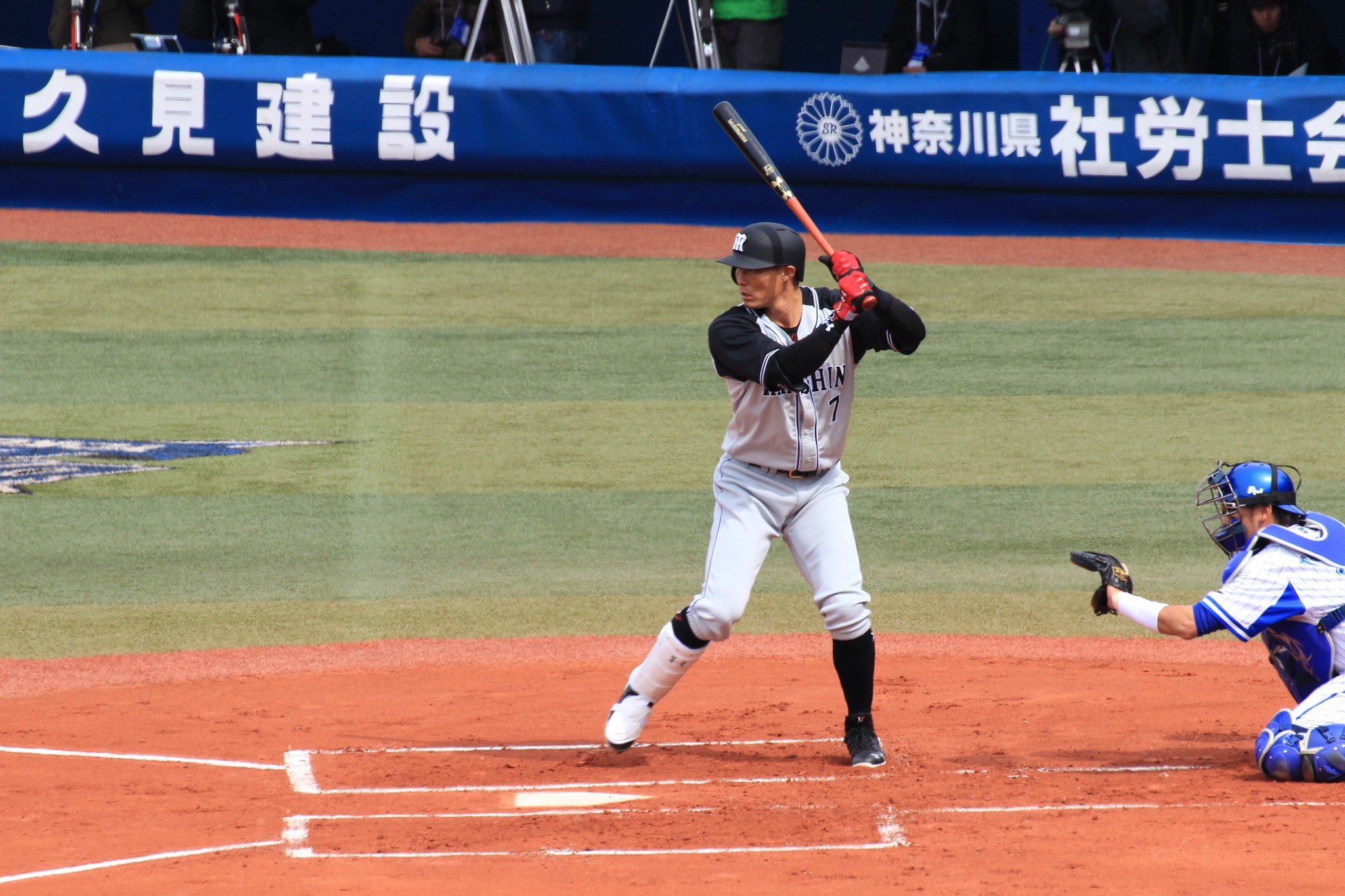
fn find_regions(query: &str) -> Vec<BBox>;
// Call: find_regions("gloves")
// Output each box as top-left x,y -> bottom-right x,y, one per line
818,250 -> 875,290
832,266 -> 873,322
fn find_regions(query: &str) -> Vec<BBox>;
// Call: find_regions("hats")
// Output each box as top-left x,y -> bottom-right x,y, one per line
716,221 -> 806,282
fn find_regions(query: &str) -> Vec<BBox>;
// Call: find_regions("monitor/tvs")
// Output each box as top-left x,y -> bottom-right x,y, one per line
129,33 -> 183,53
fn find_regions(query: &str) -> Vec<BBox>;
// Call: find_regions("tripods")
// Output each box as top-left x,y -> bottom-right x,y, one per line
1057,50 -> 1101,74
649,0 -> 724,70
463,0 -> 537,67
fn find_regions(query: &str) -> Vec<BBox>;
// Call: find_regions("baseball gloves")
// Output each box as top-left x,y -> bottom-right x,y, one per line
1070,551 -> 1132,619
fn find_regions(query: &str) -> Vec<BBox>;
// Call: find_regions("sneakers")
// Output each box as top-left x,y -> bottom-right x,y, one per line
843,711 -> 886,768
604,680 -> 654,752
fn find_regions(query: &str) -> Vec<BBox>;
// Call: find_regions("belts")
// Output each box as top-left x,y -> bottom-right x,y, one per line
749,461 -> 828,479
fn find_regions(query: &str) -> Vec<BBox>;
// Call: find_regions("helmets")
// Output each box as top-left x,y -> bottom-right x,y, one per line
1210,460 -> 1307,517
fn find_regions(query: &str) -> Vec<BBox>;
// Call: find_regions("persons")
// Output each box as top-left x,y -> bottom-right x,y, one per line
1092,459 -> 1345,778
49,0 -> 1345,75
605,221 -> 928,767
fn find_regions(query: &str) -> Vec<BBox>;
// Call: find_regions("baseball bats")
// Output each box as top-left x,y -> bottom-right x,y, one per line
715,96 -> 876,309
